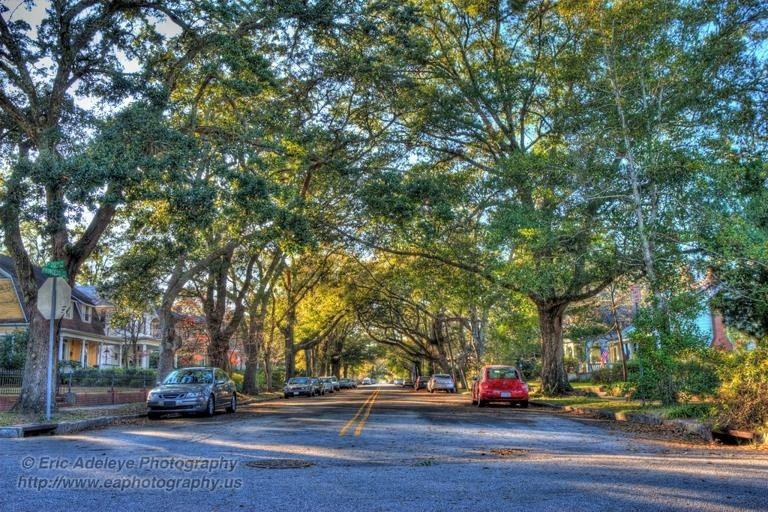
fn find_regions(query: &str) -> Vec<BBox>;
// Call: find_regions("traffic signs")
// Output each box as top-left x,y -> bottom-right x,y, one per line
46,260 -> 65,269
40,267 -> 67,278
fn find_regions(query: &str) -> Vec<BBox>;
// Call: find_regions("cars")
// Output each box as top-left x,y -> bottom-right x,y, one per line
146,365 -> 238,416
282,375 -> 358,399
394,373 -> 456,392
362,377 -> 376,384
470,364 -> 529,408
